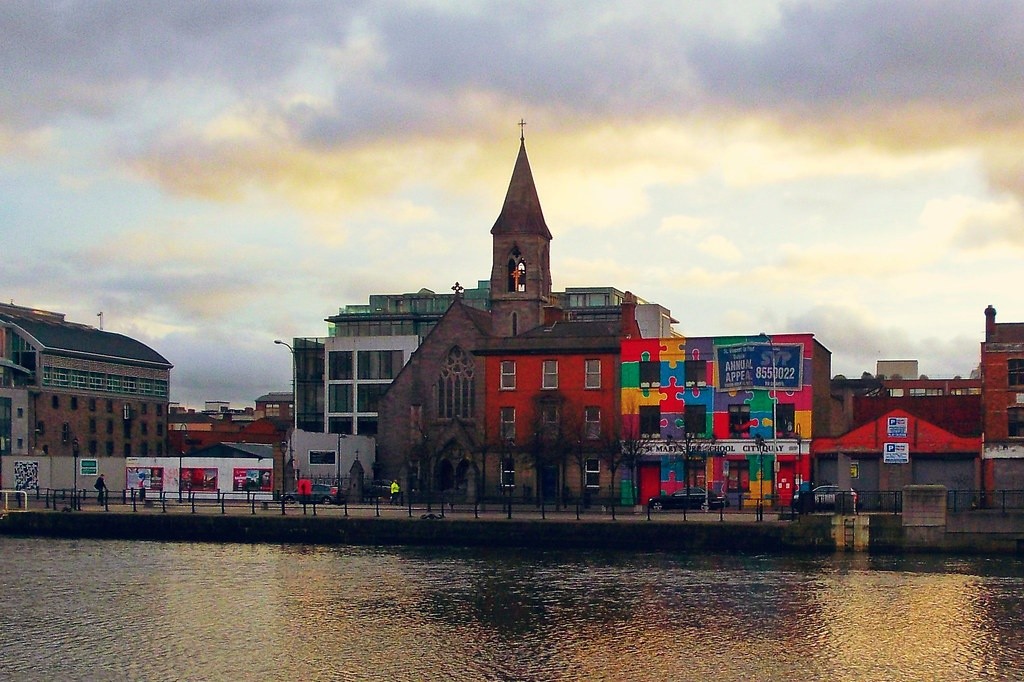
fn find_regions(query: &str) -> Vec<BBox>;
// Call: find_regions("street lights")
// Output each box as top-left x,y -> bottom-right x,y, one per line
759,332 -> 777,509
274,339 -> 295,481
280,441 -> 288,513
338,434 -> 348,504
666,433 -> 690,521
179,422 -> 188,503
794,423 -> 802,488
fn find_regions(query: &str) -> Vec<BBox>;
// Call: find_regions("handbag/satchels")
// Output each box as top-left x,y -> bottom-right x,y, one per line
94,484 -> 98,489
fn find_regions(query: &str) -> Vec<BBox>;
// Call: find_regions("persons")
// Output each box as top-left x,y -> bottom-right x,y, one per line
137,473 -> 146,488
389,480 -> 400,505
251,470 -> 271,486
95,474 -> 108,506
16,481 -> 22,508
851,488 -> 859,513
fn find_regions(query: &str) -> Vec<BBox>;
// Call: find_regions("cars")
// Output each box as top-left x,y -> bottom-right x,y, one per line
281,484 -> 340,505
648,486 -> 725,511
793,485 -> 858,509
372,479 -> 393,497
